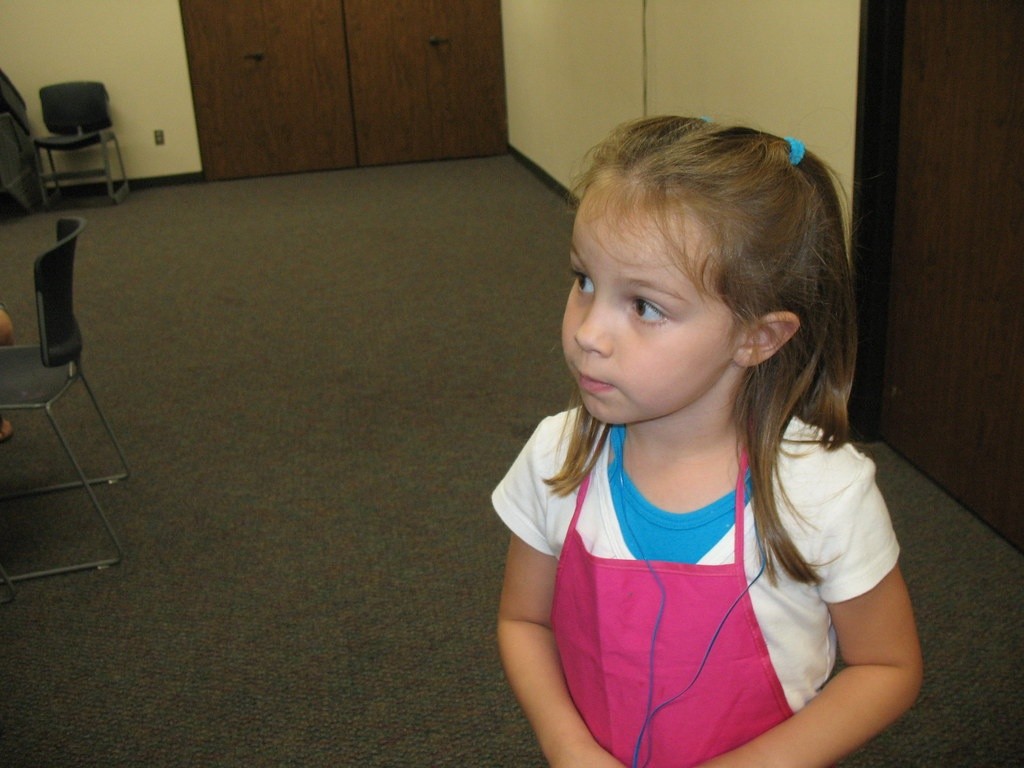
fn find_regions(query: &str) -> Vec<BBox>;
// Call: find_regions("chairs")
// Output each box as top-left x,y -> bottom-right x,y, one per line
0,216 -> 131,584
31,80 -> 129,204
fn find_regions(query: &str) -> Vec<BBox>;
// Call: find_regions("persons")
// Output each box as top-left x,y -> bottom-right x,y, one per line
489,113 -> 925,768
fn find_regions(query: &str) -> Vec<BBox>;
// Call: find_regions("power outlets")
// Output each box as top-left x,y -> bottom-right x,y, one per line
155,130 -> 164,144
